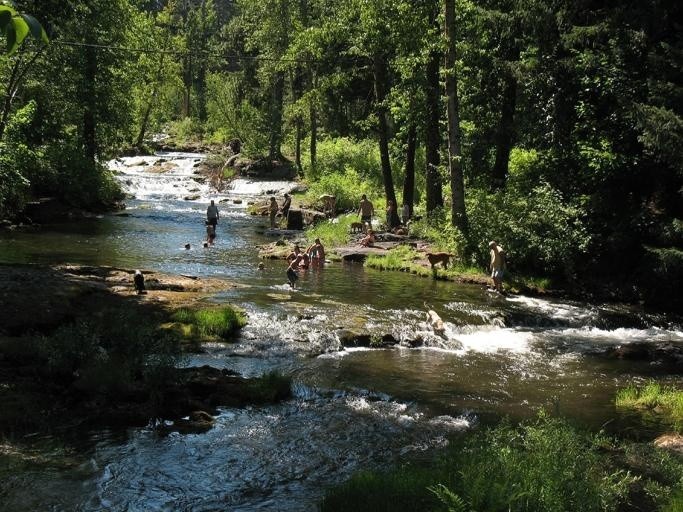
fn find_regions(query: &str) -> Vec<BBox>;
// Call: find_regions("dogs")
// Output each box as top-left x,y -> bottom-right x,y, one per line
350,222 -> 363,234
423,301 -> 446,336
425,253 -> 455,270
317,194 -> 337,209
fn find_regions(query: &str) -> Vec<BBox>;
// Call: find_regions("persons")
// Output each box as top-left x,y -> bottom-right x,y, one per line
356,194 -> 375,247
402,201 -> 409,224
269,197 -> 278,228
319,194 -> 336,213
185,243 -> 190,249
281,194 -> 291,221
257,262 -> 267,272
286,238 -> 325,289
204,200 -> 219,247
386,200 -> 393,225
488,241 -> 504,293
